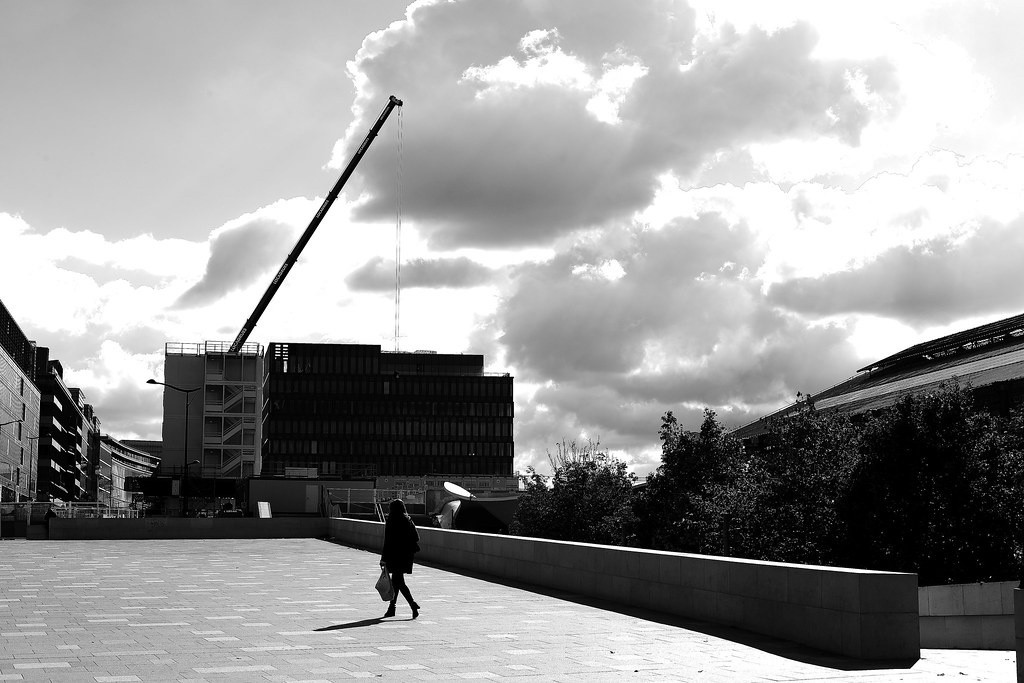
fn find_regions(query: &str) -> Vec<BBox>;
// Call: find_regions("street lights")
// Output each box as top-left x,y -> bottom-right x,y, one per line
145,379 -> 202,517
26,433 -> 51,501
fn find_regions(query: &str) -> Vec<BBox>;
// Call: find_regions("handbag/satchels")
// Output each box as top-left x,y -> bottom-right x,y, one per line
375,561 -> 395,601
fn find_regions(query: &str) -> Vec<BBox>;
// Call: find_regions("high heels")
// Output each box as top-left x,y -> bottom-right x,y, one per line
385,607 -> 396,617
411,602 -> 420,618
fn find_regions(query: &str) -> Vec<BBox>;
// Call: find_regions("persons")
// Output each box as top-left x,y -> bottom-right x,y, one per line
380,499 -> 420,619
